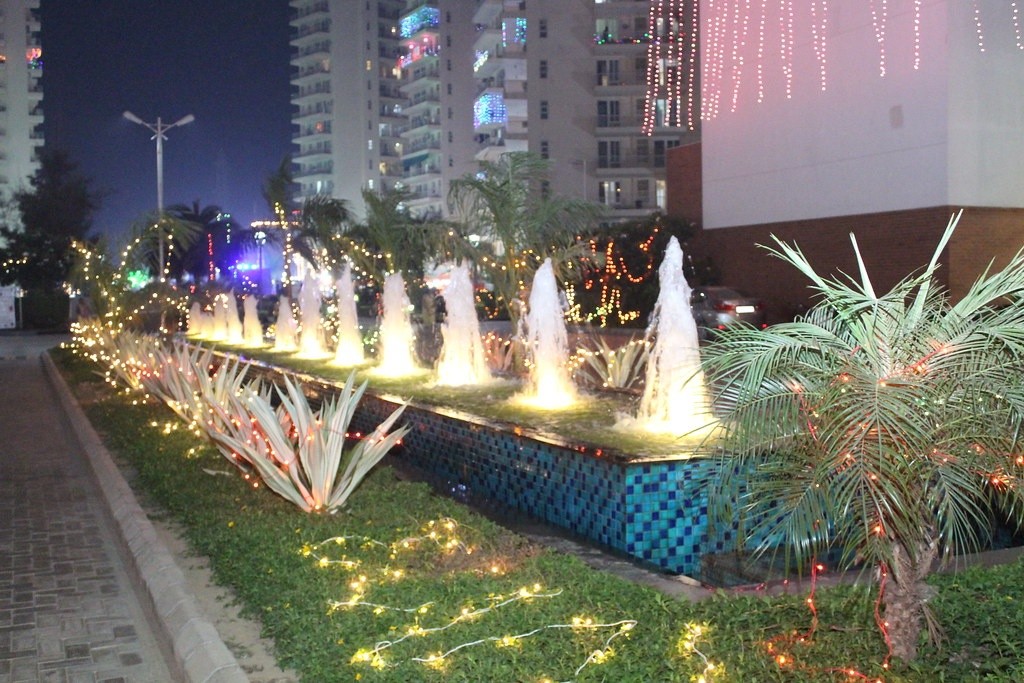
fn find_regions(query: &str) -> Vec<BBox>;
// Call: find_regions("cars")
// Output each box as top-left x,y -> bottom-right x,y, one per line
691,285 -> 767,346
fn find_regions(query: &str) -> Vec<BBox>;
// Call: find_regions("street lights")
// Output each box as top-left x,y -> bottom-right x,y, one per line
122,107 -> 196,284
